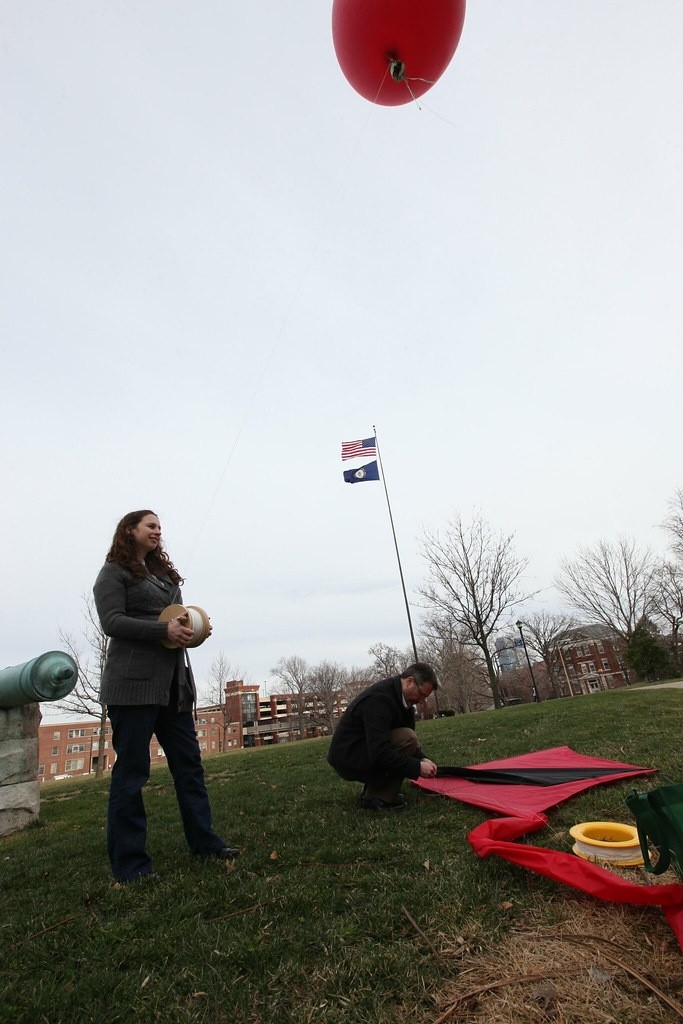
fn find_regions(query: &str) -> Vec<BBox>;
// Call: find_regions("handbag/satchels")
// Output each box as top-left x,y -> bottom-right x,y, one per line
625,782 -> 683,883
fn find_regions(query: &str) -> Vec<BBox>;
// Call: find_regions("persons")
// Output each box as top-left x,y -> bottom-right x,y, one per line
91,510 -> 237,887
328,662 -> 438,812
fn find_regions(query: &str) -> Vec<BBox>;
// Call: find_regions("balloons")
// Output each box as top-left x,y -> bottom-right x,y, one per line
332,0 -> 466,107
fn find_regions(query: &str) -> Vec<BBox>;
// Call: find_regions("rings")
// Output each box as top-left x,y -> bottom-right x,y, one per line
177,616 -> 182,622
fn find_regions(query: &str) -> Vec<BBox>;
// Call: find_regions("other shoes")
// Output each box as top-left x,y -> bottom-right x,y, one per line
207,848 -> 240,860
360,794 -> 407,810
119,874 -> 161,886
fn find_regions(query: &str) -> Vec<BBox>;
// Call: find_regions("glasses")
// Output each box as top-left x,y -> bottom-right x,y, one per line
414,680 -> 428,700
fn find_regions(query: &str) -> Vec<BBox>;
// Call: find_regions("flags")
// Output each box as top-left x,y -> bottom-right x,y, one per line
341,436 -> 380,485
